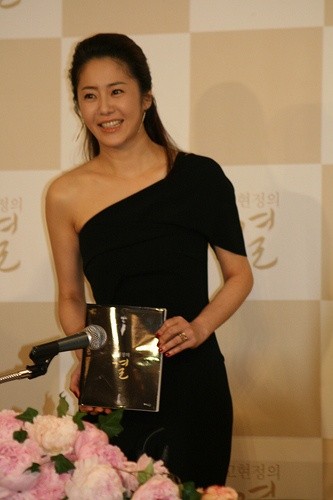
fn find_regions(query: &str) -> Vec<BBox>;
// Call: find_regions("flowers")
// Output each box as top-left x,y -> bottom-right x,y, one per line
0,391 -> 242,500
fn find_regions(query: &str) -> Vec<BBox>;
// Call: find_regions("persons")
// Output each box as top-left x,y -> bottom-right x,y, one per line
44,33 -> 254,493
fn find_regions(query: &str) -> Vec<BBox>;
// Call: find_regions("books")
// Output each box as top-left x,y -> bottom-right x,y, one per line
77,303 -> 167,413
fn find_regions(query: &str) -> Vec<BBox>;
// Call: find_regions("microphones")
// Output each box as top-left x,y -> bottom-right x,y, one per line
29,324 -> 107,364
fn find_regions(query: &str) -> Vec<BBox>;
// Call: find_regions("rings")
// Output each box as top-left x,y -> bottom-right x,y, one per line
179,332 -> 189,342
